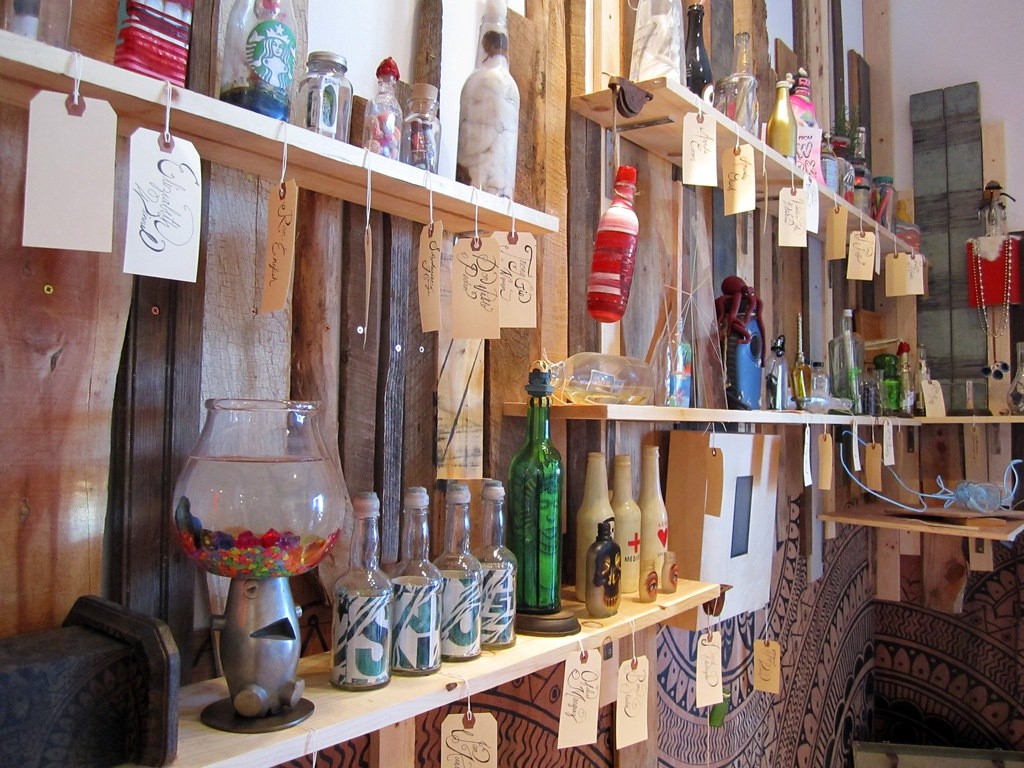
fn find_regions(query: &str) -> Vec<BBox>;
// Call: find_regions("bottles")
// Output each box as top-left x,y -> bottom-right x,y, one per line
219,0 -> 441,173
1007,342 -> 1024,416
329,479 -> 517,691
529,352 -> 657,406
828,309 -> 929,416
507,361 -> 563,614
849,127 -> 871,216
766,81 -> 797,167
733,32 -> 758,137
456,0 -> 520,200
629,0 -> 686,86
868,176 -> 897,234
788,352 -> 811,409
810,362 -> 830,397
586,165 -> 639,324
763,339 -> 790,412
655,317 -> 692,408
685,4 -> 715,108
575,444 -> 679,618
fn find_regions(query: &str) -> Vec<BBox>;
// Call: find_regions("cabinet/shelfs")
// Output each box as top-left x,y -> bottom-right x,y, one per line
0,27 -> 1024,768
